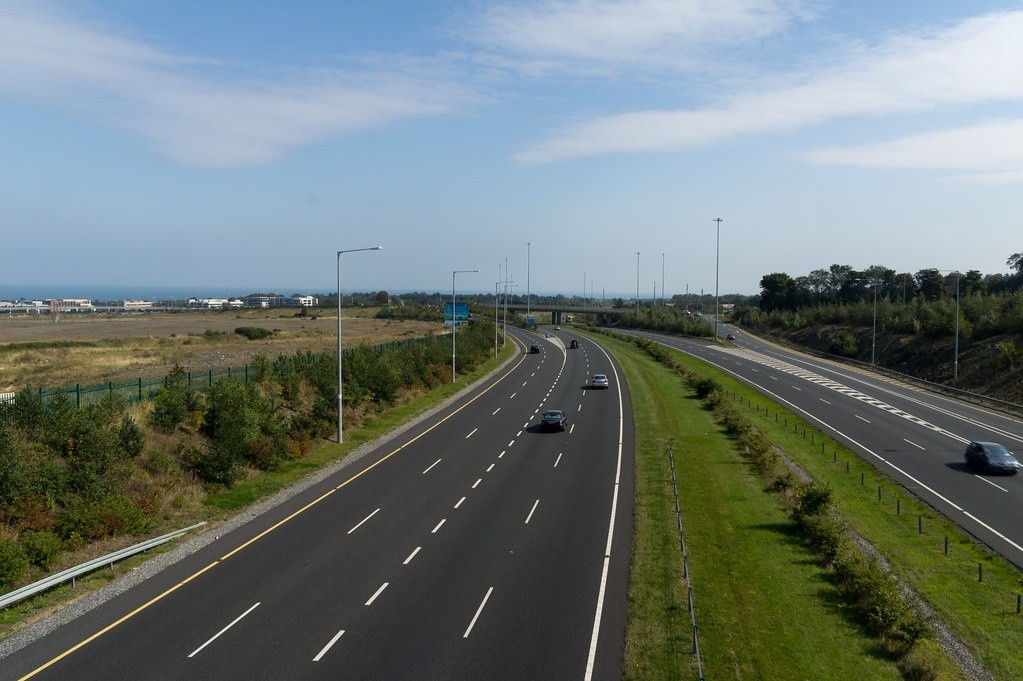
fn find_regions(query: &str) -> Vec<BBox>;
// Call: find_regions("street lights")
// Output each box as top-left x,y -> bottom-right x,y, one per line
855,278 -> 878,364
503,284 -> 519,348
452,270 -> 481,383
928,267 -> 961,386
336,246 -> 384,445
494,280 -> 515,359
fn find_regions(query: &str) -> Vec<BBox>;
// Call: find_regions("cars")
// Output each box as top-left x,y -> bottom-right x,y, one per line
541,409 -> 568,431
684,310 -> 692,315
964,440 -> 1022,477
694,311 -> 702,317
571,339 -> 579,348
554,326 -> 561,331
531,344 -> 540,354
727,333 -> 736,339
591,374 -> 609,389
